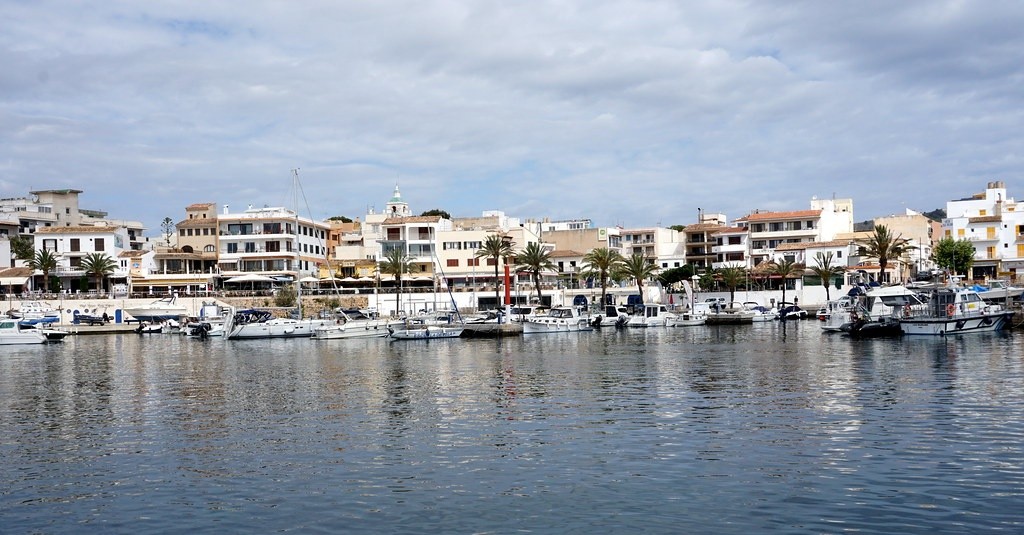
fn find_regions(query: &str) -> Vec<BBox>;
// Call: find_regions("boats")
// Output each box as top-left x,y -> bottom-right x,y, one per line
898,246 -> 1015,335
0,285 -> 979,341
973,277 -> 1024,330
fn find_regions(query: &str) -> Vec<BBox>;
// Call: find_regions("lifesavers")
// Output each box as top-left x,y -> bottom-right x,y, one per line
904,304 -> 911,316
946,303 -> 955,317
851,314 -> 858,323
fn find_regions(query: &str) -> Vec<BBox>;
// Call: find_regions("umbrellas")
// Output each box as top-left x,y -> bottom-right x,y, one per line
293,277 -> 432,289
223,274 -> 275,291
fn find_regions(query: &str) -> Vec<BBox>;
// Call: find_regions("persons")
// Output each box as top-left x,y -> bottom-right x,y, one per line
179,289 -> 186,298
794,296 -> 798,306
